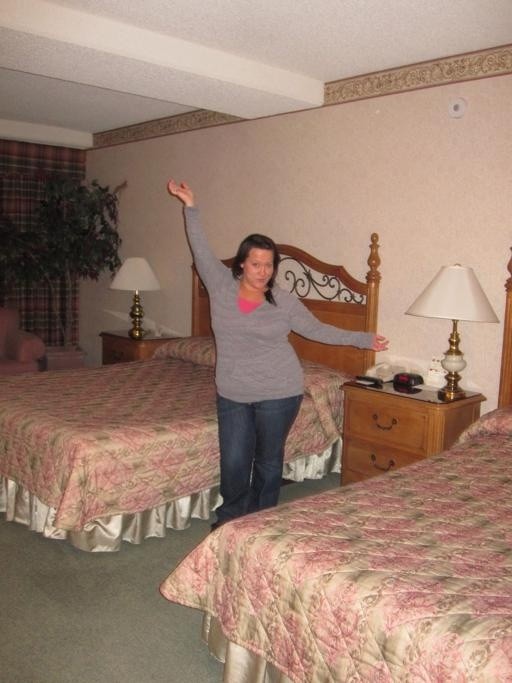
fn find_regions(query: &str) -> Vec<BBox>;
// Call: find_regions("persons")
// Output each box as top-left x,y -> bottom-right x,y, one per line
166,178 -> 390,534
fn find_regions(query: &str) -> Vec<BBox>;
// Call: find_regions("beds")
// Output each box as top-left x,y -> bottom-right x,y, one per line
0,232 -> 381,552
162,230 -> 512,683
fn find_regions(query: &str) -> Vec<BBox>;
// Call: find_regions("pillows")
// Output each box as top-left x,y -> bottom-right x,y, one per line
151,335 -> 216,367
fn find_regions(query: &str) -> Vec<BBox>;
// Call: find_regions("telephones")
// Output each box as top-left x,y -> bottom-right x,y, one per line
362,361 -> 405,382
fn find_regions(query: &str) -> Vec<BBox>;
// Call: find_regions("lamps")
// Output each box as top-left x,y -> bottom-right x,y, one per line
405,263 -> 500,402
109,257 -> 162,338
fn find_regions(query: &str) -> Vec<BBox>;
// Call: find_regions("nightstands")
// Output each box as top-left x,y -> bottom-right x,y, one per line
340,382 -> 486,487
102,331 -> 180,367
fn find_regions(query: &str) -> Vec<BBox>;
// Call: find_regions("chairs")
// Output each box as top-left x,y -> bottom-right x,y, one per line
0,308 -> 46,372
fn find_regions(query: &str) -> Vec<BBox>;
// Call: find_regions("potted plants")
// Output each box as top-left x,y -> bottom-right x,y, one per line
0,173 -> 121,372
